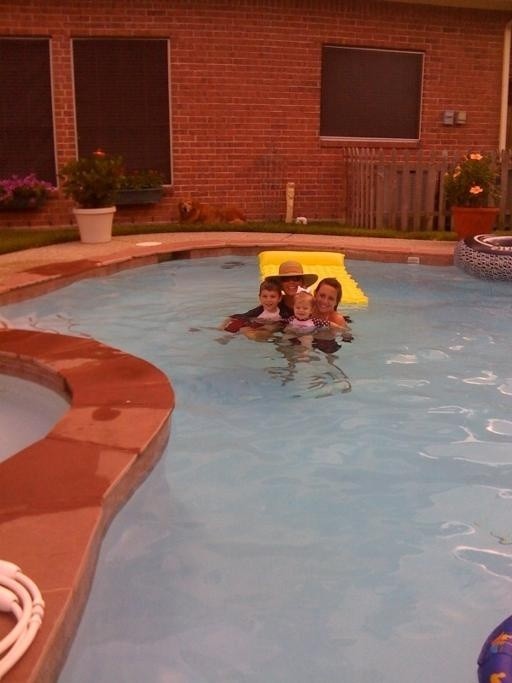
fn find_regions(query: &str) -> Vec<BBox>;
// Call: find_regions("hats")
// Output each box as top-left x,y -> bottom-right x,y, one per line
265,260 -> 318,289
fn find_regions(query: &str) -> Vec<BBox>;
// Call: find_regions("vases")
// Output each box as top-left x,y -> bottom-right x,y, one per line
73,205 -> 117,244
449,204 -> 499,241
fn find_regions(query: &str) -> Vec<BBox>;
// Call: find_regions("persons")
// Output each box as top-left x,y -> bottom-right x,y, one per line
244,262 -> 346,329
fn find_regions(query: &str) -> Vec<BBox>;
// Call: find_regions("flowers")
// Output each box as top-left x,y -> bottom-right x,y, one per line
442,150 -> 503,209
58,148 -> 165,208
1,173 -> 56,211
